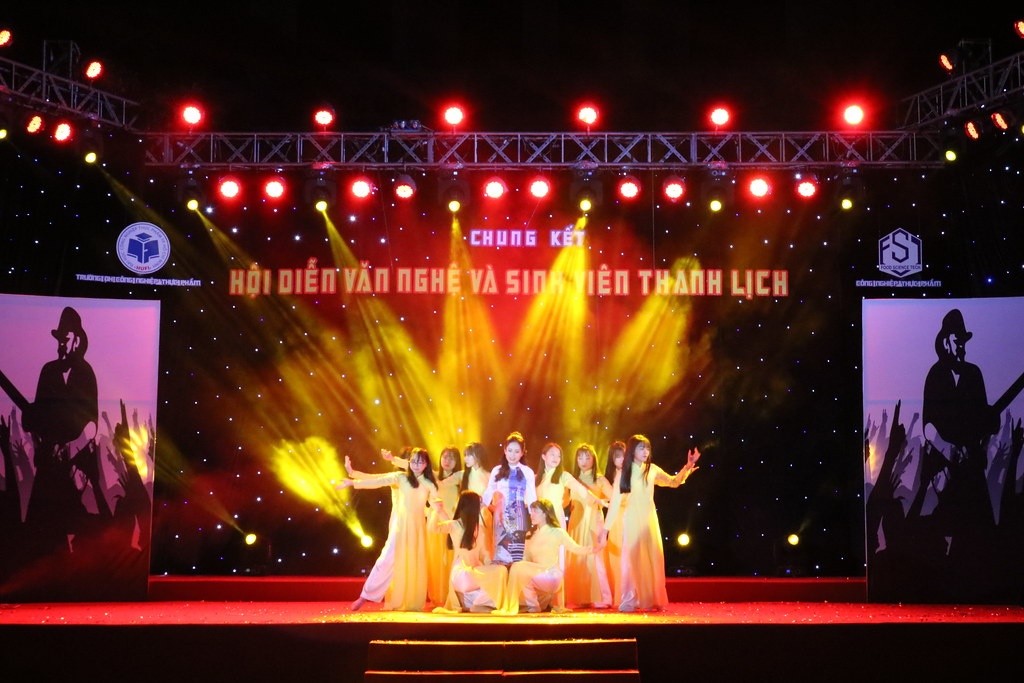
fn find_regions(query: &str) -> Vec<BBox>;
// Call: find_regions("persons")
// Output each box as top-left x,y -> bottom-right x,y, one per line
493,497 -> 605,613
333,431 -> 700,613
426,491 -> 507,613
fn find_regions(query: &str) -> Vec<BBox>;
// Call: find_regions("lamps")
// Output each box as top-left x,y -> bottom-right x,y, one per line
699,178 -> 737,213
964,99 -> 1024,141
241,532 -> 272,562
568,172 -> 603,211
667,532 -> 697,573
304,176 -> 336,213
435,174 -> 471,212
174,174 -> 206,212
938,40 -> 971,71
778,531 -> 804,567
